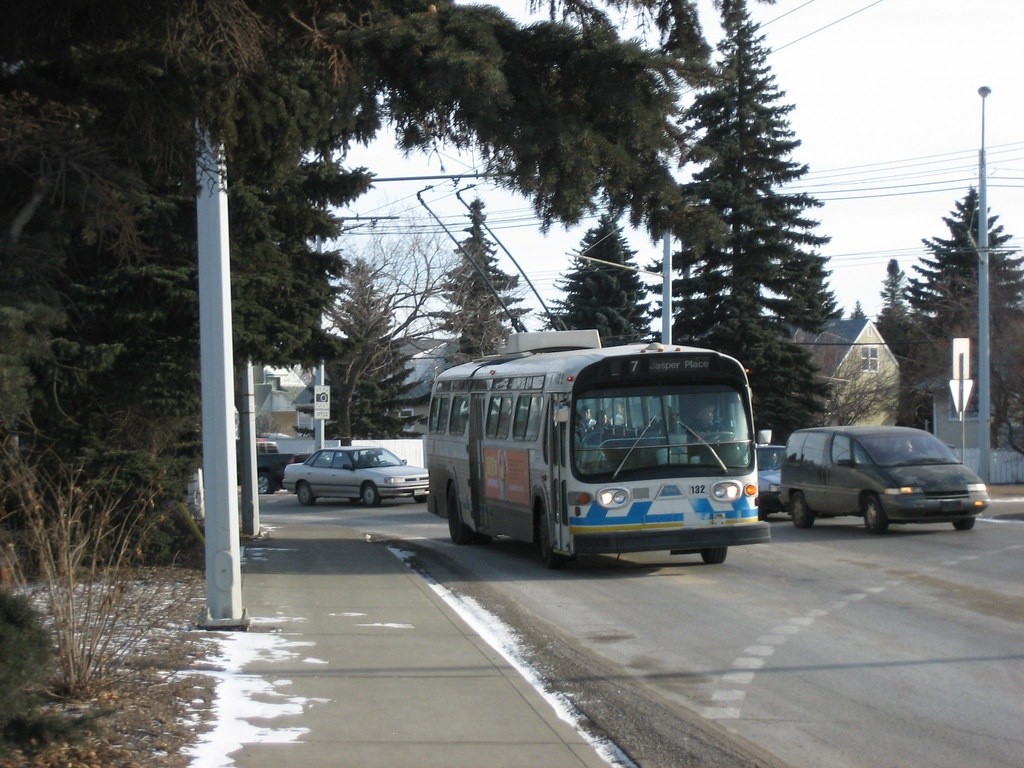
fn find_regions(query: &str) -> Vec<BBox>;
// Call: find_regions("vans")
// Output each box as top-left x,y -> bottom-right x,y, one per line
778,427 -> 989,533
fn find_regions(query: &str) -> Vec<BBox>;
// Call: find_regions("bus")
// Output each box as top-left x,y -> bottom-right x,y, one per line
422,328 -> 773,569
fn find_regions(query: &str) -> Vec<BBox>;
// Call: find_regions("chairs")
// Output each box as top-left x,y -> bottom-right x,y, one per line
443,413 -> 653,460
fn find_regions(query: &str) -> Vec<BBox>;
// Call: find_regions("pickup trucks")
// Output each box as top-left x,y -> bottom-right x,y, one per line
236,439 -> 313,495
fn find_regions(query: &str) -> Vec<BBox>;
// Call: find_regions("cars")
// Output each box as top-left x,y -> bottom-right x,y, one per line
281,447 -> 430,509
754,442 -> 788,520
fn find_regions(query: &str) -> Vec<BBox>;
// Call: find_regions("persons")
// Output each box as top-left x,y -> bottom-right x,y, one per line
611,403 -> 625,426
688,401 -> 736,443
579,406 -> 597,432
366,451 -> 376,466
589,410 -> 612,431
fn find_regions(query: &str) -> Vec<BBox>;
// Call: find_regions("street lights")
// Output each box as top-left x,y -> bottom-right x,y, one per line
978,87 -> 992,485
